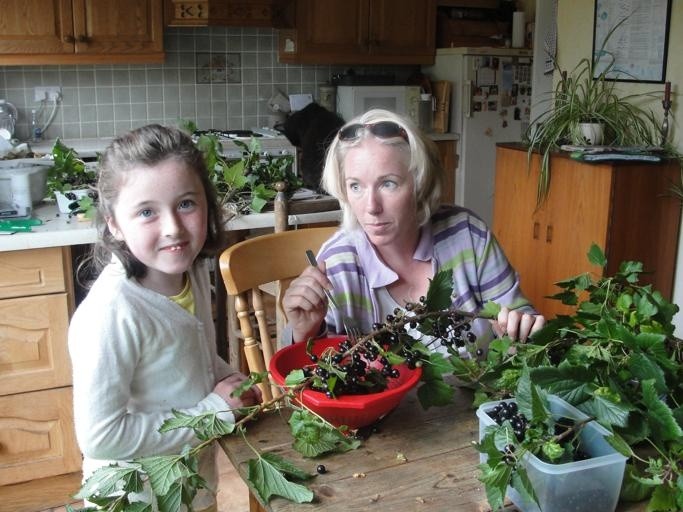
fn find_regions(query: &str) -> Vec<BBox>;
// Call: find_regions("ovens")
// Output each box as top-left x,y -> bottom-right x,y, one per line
213,146 -> 297,182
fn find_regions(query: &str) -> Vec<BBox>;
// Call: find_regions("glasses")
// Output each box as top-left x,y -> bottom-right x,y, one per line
336,120 -> 411,146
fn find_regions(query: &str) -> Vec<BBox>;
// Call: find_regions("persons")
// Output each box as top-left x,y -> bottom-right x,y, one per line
67,122 -> 265,511
280,106 -> 548,390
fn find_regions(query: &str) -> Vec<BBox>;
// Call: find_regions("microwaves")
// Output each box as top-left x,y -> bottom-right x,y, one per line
336,86 -> 421,128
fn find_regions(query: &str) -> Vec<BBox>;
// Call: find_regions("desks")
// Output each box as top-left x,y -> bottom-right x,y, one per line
215,347 -> 682,511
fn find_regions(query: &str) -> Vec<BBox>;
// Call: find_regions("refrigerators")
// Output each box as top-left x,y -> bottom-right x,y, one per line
435,47 -> 533,231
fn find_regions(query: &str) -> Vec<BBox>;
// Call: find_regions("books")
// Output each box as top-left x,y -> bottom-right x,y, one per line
559,141 -> 665,164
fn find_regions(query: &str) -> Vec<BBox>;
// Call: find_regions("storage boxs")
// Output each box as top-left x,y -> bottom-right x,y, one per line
474,393 -> 631,512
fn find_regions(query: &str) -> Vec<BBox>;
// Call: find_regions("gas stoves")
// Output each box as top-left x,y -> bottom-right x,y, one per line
190,126 -> 287,144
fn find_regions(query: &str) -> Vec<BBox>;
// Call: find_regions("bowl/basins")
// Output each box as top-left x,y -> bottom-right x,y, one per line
269,334 -> 422,437
53,189 -> 92,214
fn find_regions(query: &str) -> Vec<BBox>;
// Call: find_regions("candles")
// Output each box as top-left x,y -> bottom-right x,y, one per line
666,80 -> 670,103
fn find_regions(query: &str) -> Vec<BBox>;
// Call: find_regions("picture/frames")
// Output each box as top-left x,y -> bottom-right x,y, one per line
591,0 -> 673,83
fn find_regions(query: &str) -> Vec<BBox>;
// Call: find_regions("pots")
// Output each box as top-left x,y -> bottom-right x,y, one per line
0,158 -> 50,206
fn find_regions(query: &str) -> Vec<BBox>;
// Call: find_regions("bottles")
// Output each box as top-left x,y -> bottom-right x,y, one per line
418,93 -> 433,133
10,173 -> 32,219
320,86 -> 334,112
512,11 -> 525,49
32,109 -> 42,143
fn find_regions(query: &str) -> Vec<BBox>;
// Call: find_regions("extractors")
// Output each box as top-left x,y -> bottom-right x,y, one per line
167,19 -> 281,27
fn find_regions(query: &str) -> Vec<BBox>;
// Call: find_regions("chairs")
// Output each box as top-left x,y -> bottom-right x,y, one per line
226,181 -> 344,375
218,227 -> 344,409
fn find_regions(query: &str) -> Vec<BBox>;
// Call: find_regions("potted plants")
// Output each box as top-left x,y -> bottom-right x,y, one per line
522,8 -> 683,209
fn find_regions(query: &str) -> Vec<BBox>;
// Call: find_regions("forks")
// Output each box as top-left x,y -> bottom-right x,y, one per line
306,250 -> 362,342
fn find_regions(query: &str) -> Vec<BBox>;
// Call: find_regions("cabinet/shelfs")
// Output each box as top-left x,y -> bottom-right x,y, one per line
425,132 -> 459,210
0,185 -> 342,512
494,141 -> 683,330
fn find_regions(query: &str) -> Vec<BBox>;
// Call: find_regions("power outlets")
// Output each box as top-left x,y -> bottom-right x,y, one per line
35,87 -> 62,104
